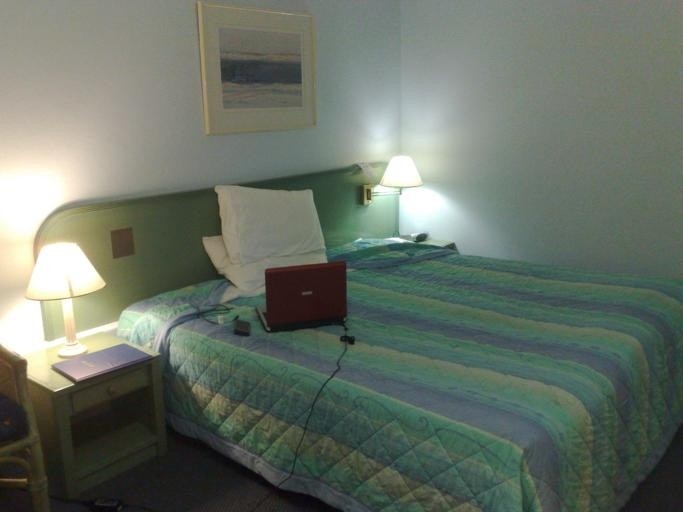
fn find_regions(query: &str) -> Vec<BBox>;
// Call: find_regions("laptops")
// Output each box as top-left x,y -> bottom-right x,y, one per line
255,259 -> 348,332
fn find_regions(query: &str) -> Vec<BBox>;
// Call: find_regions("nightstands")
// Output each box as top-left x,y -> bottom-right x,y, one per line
16,337 -> 167,503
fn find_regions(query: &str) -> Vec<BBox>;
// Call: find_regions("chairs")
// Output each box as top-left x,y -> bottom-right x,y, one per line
0,344 -> 48,512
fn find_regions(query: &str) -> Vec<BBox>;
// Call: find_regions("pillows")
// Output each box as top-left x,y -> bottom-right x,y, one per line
203,184 -> 329,303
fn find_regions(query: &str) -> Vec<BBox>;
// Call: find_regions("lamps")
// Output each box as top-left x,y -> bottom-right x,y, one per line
24,242 -> 108,360
363,156 -> 422,208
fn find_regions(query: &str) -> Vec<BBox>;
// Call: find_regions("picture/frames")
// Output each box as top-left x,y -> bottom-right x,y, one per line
197,1 -> 317,136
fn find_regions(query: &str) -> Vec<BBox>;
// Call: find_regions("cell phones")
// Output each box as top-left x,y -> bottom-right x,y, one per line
234,319 -> 250,335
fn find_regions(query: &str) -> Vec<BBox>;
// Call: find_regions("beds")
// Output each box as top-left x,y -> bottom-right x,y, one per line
32,159 -> 682,512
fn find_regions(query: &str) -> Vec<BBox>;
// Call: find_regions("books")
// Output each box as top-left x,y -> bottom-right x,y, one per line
49,342 -> 152,382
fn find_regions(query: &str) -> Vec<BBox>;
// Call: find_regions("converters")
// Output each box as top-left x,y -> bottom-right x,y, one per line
91,497 -> 121,512
